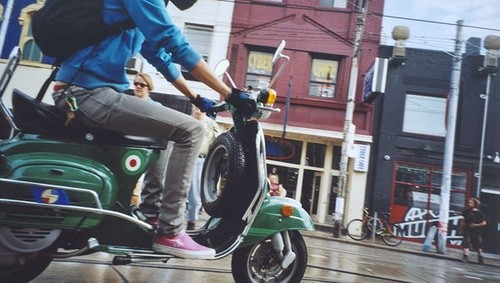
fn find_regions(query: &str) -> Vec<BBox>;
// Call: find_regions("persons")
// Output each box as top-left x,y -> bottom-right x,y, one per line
268,171 -> 287,199
51,0 -> 251,260
125,71 -> 161,210
178,100 -> 217,232
460,197 -> 488,265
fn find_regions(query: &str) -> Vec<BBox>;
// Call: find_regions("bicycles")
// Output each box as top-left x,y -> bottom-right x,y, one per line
346,206 -> 404,246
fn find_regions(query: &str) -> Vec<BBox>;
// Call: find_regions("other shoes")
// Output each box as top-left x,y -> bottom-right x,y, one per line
153,231 -> 216,260
478,256 -> 483,264
187,222 -> 194,230
462,256 -> 469,263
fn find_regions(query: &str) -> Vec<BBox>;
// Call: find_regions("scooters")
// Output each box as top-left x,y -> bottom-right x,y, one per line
0,38 -> 314,283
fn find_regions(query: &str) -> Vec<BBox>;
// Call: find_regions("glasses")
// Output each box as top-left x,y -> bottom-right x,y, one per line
134,82 -> 148,87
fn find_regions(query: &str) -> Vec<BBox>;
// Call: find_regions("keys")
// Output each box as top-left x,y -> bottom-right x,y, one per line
62,111 -> 75,127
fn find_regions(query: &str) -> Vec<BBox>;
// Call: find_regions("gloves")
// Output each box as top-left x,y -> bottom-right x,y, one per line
225,88 -> 256,118
191,94 -> 217,117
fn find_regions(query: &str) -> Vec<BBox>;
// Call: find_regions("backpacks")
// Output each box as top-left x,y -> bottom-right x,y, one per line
32,0 -> 136,59
